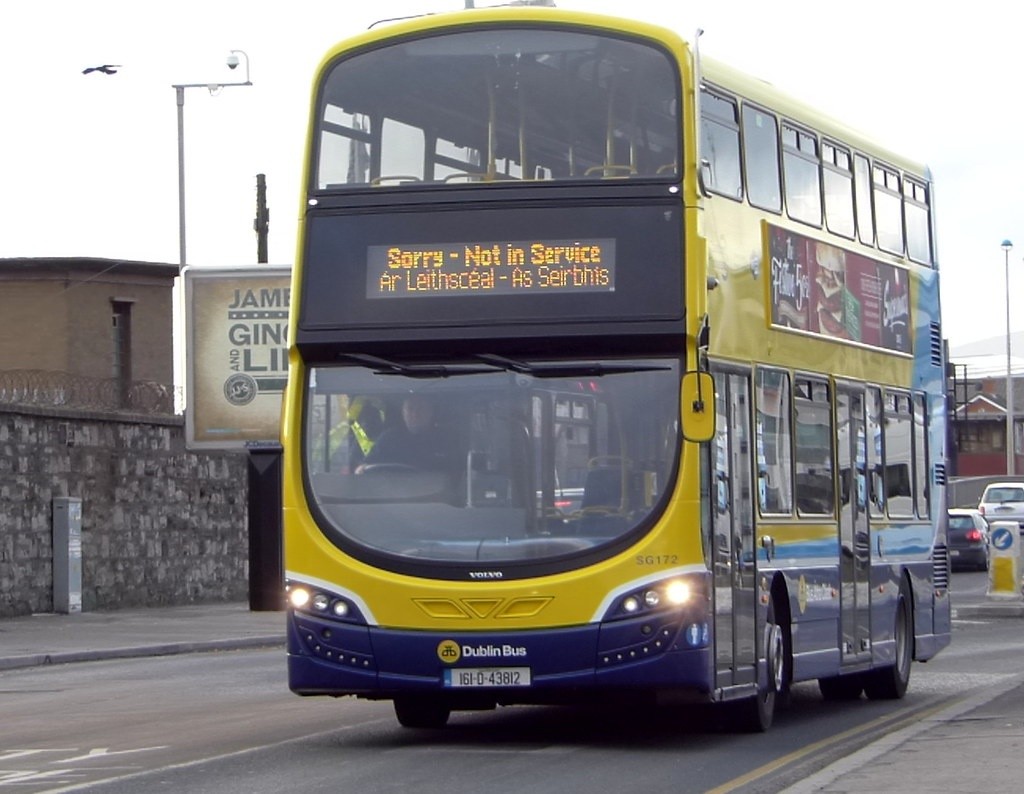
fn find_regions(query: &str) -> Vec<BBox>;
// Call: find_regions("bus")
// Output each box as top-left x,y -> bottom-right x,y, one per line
276,0 -> 958,734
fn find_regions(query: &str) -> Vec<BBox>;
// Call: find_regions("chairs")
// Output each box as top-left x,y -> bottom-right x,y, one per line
369,169 -> 668,513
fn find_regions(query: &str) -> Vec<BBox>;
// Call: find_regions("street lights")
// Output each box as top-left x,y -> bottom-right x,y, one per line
171,49 -> 255,264
1001,237 -> 1017,475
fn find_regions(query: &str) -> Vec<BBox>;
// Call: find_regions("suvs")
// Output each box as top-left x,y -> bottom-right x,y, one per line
946,509 -> 991,572
976,482 -> 1024,532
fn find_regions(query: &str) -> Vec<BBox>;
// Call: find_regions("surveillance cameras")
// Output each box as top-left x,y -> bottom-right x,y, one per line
707,276 -> 718,290
227,55 -> 239,69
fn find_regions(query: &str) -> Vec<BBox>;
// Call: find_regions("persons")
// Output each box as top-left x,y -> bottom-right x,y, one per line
353,391 -> 463,476
